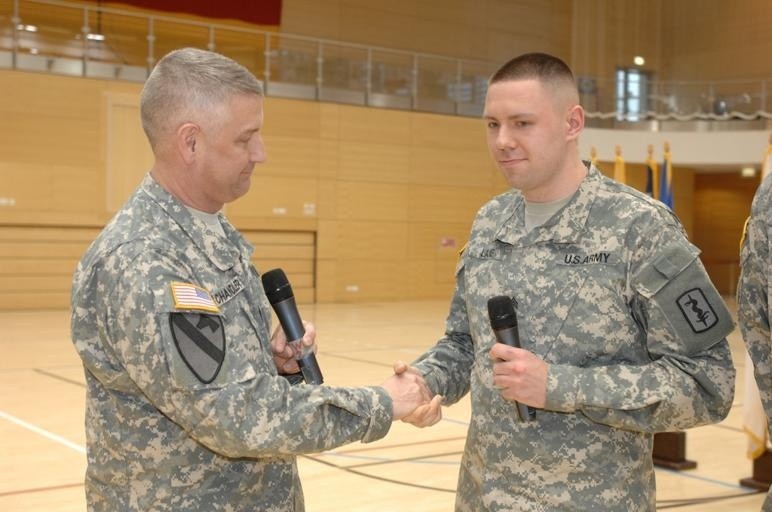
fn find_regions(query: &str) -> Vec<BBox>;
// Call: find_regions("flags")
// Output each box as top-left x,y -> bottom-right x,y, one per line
742,346 -> 768,459
614,153 -> 625,184
660,153 -> 672,207
170,281 -> 218,312
646,156 -> 657,197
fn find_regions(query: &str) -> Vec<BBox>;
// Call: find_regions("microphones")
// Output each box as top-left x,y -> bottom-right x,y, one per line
260,269 -> 326,386
486,295 -> 538,424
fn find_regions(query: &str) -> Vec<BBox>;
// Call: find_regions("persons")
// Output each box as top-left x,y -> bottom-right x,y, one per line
69,46 -> 432,512
393,52 -> 735,511
734,170 -> 771,512
